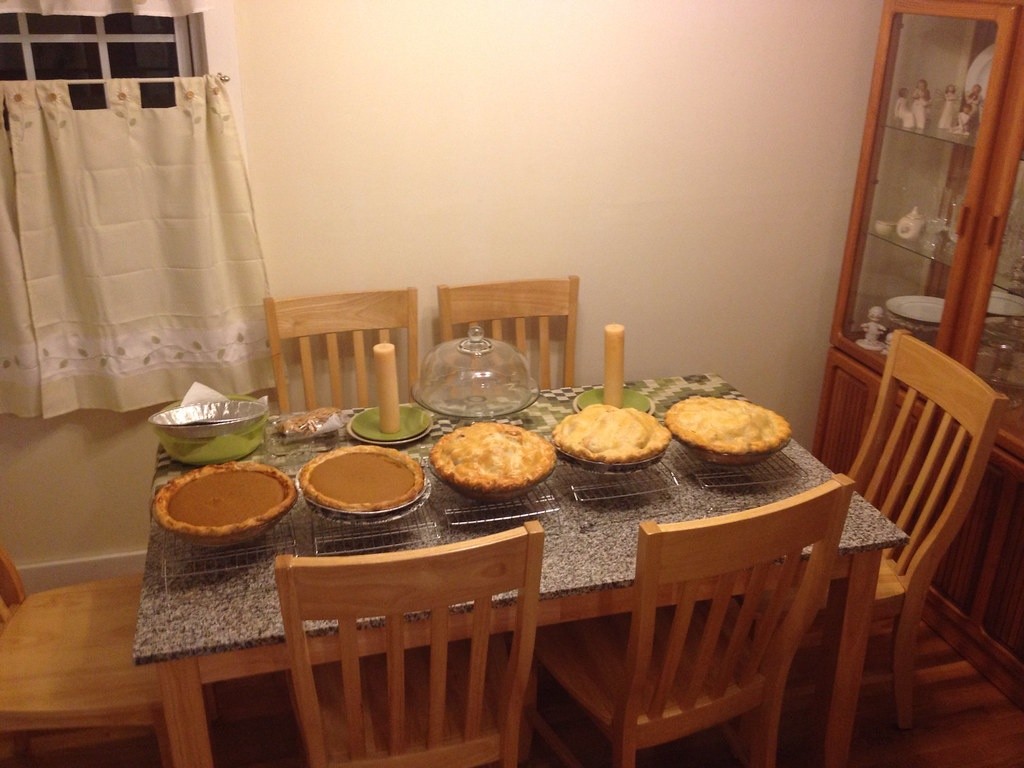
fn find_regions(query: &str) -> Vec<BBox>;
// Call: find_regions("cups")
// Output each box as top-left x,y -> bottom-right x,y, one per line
925,184 -> 947,233
947,196 -> 966,243
875,220 -> 895,235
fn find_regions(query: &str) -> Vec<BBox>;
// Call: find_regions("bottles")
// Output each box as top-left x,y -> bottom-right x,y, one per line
897,206 -> 926,243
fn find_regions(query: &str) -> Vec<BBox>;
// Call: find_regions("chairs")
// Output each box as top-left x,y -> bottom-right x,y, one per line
753,326 -> 1009,731
0,542 -> 172,768
522,473 -> 857,768
266,283 -> 419,415
272,519 -> 546,768
437,272 -> 579,392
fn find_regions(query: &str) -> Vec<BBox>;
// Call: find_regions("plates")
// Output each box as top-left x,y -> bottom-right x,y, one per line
886,294 -> 947,321
349,408 -> 431,447
411,377 -> 540,419
575,387 -> 653,421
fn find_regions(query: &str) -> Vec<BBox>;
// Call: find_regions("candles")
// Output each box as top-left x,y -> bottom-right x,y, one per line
603,321 -> 624,409
371,340 -> 399,433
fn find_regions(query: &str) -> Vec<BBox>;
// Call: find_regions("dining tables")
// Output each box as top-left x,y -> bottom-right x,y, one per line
128,373 -> 910,768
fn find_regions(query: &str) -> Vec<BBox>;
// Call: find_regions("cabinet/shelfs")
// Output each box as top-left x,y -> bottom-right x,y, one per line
830,0 -> 1024,462
808,346 -> 1024,707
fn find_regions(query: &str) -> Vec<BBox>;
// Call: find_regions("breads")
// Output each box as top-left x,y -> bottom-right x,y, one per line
152,461 -> 297,545
427,421 -> 557,497
665,395 -> 791,466
551,403 -> 672,472
299,445 -> 428,521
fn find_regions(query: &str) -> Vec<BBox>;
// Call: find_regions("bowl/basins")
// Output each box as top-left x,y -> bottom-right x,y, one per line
429,452 -> 556,496
665,436 -> 795,464
296,465 -> 427,515
150,394 -> 266,460
552,436 -> 668,471
